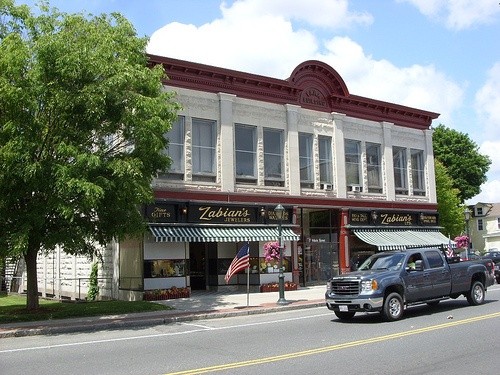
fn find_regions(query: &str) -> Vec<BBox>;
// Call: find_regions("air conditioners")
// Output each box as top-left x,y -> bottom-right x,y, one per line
352,186 -> 360,192
324,184 -> 332,191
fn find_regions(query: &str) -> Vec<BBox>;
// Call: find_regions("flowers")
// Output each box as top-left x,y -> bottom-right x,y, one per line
454,236 -> 469,248
145,286 -> 188,295
264,242 -> 285,262
262,281 -> 296,287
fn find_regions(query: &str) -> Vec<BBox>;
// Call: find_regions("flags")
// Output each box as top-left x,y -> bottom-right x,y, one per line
223,244 -> 249,283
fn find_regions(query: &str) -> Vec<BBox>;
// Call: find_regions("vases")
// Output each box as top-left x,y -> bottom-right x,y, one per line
261,287 -> 298,292
144,295 -> 190,300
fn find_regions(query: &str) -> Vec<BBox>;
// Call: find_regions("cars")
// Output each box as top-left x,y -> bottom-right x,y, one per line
483,252 -> 500,282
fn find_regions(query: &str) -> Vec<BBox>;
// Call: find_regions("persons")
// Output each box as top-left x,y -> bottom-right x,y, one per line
407,257 -> 416,270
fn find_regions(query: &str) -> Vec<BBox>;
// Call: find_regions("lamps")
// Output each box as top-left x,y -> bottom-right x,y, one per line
419,212 -> 424,221
372,211 -> 377,220
261,206 -> 265,216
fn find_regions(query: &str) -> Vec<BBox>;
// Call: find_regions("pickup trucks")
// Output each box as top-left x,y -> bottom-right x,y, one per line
326,247 -> 495,320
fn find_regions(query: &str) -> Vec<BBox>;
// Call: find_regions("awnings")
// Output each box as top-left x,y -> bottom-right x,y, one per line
148,226 -> 300,243
352,229 -> 457,251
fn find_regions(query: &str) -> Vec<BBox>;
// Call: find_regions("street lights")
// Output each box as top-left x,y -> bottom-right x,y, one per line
464,207 -> 471,260
274,204 -> 288,305
